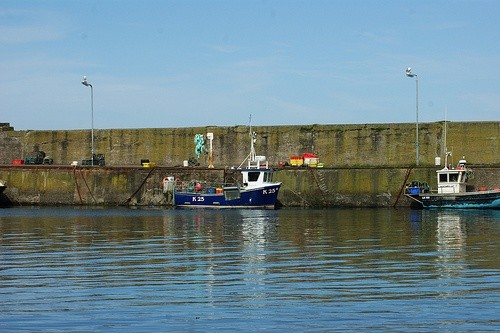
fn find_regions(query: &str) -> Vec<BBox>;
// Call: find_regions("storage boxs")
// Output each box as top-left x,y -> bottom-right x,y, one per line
142,163 -> 155,167
12,151 -> 53,165
82,154 -> 105,166
290,153 -> 323,168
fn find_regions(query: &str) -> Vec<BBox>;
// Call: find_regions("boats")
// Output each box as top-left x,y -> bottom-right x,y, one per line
404,153 -> 500,209
172,169 -> 282,209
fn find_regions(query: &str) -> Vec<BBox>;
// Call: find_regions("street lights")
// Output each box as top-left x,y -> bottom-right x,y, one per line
81,76 -> 95,166
405,66 -> 419,166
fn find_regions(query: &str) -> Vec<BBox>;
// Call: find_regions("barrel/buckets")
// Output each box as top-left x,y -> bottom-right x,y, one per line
207,187 -> 215,194
434,157 -> 441,165
183,160 -> 188,166
215,189 -> 223,194
